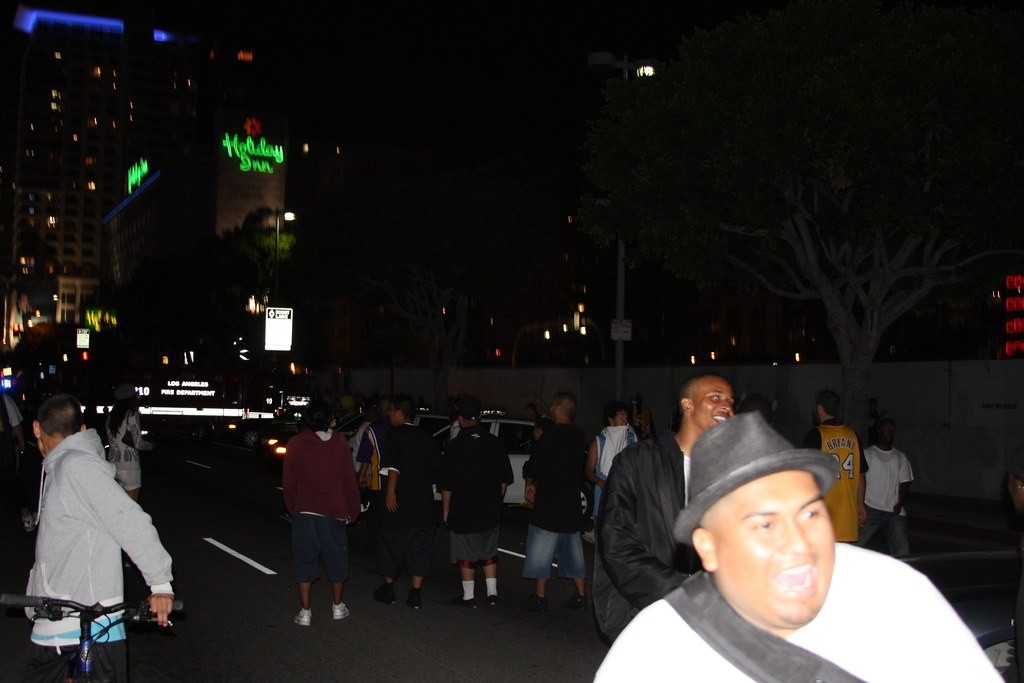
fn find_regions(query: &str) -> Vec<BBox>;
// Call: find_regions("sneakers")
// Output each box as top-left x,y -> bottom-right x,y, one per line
332,603 -> 349,620
295,609 -> 312,626
24,512 -> 38,532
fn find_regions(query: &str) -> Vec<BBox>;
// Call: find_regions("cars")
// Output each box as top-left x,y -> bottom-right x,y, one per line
133,389 -> 596,523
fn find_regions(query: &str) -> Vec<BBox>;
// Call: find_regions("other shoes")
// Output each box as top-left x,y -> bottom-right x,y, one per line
406,595 -> 421,609
485,595 -> 499,607
450,596 -> 477,608
527,593 -> 548,611
563,594 -> 587,608
381,585 -> 396,604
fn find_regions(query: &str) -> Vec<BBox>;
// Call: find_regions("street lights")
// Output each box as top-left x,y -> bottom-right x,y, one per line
274,208 -> 296,304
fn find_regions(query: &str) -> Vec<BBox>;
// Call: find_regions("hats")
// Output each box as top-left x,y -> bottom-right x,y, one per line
457,397 -> 481,419
115,384 -> 137,400
674,409 -> 838,543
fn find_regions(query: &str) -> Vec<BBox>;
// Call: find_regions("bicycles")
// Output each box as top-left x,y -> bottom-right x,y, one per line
0,593 -> 189,682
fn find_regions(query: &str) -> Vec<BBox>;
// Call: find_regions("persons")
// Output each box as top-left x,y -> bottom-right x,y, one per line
352,397 -> 392,569
522,392 -> 590,612
521,403 -> 545,445
869,397 -> 880,445
621,379 -> 658,443
591,372 -> 733,645
584,400 -> 641,535
591,406 -> 1006,683
738,394 -> 774,428
105,384 -> 157,568
24,393 -> 174,683
802,391 -> 870,544
440,397 -> 514,608
1007,439 -> 1024,683
282,400 -> 360,627
373,395 -> 444,609
850,418 -> 915,562
0,393 -> 39,532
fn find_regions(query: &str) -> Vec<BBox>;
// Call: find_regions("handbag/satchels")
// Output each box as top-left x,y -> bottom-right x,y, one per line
121,411 -> 135,447
